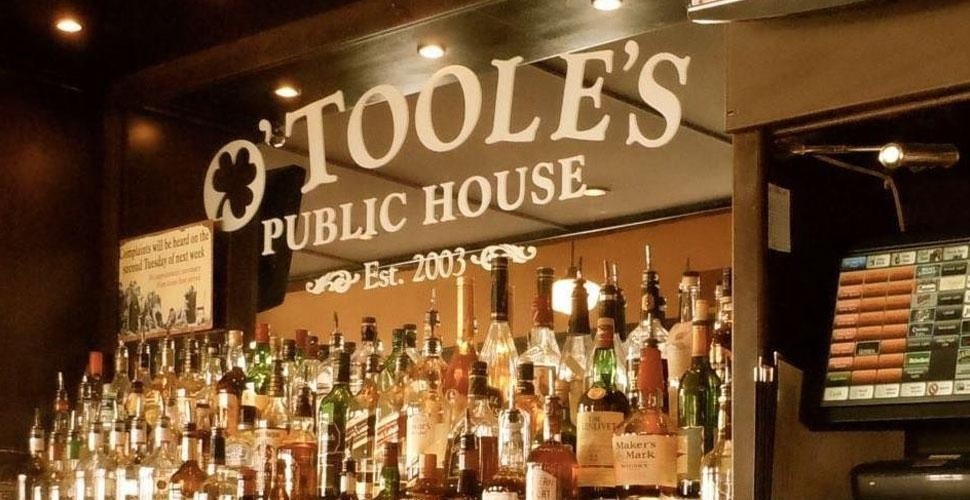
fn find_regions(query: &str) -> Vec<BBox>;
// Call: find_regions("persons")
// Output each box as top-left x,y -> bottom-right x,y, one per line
125,282 -> 196,333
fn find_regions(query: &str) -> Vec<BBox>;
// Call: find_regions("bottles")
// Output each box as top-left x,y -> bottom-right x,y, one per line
18,241 -> 731,500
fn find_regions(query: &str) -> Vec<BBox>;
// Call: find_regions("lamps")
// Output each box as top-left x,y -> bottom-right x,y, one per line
548,237 -> 601,319
791,136 -> 962,232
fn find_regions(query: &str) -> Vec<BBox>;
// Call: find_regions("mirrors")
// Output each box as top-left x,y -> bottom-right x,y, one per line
95,1 -> 732,350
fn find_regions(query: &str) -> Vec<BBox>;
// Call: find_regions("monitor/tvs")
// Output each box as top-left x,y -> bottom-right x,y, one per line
798,230 -> 970,500
